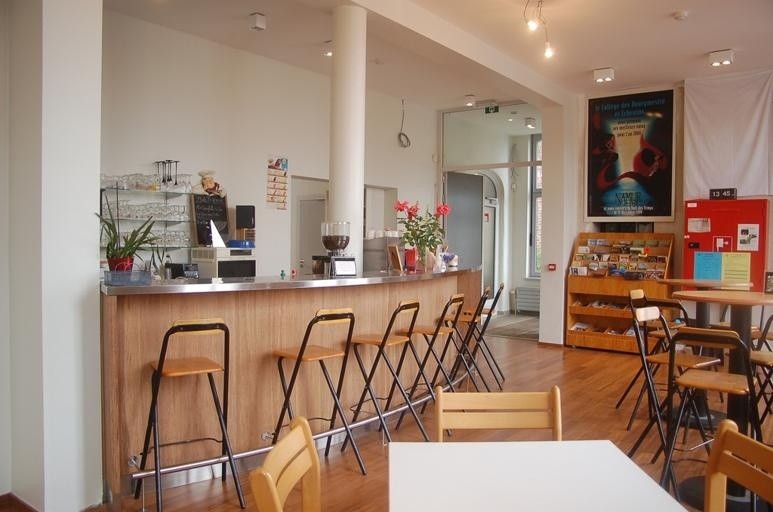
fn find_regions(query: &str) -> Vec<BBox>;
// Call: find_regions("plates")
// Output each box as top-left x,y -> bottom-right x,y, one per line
565,232 -> 674,354
99,187 -> 192,251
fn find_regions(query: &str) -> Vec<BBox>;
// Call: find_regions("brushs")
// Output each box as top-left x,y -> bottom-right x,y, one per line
320,220 -> 351,275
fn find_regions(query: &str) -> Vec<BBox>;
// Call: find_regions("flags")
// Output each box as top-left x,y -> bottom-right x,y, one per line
416,246 -> 427,273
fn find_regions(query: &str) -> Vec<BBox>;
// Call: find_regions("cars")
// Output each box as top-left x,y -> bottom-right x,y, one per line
101,173 -> 160,191
119,229 -> 190,248
367,230 -> 399,240
448,255 -> 458,267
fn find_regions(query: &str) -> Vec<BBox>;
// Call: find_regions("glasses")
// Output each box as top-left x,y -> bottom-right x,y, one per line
526,118 -> 535,129
522,1 -> 553,59
594,69 -> 615,83
709,49 -> 733,66
322,42 -> 332,56
248,13 -> 266,31
463,95 -> 475,106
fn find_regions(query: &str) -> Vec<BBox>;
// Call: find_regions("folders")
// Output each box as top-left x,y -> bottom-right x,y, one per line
516,286 -> 540,314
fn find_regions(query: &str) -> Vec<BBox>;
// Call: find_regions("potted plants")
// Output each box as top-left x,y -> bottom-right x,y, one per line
584,85 -> 677,224
387,246 -> 402,272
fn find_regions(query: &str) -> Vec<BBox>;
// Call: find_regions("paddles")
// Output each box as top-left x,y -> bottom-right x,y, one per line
483,317 -> 538,339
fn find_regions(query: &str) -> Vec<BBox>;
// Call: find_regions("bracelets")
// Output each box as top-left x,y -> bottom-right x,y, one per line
227,239 -> 255,248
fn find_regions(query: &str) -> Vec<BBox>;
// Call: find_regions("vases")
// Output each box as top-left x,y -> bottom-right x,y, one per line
394,200 -> 450,249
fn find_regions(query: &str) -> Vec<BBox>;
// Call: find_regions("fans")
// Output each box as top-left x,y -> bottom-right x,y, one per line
191,194 -> 230,246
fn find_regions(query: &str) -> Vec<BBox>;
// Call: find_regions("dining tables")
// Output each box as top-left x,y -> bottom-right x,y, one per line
94,182 -> 158,270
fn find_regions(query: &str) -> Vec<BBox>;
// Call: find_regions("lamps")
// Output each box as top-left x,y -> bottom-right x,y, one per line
395,294 -> 467,435
248,417 -> 319,512
126,318 -> 246,511
273,307 -> 368,474
342,298 -> 429,449
435,281 -> 505,391
621,289 -> 773,512
434,383 -> 561,442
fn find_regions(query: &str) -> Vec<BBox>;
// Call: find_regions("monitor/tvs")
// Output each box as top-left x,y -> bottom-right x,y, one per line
569,238 -> 670,338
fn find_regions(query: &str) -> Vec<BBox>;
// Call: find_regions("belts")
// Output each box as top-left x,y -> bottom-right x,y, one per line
172,173 -> 193,194
154,159 -> 181,193
102,199 -> 189,222
